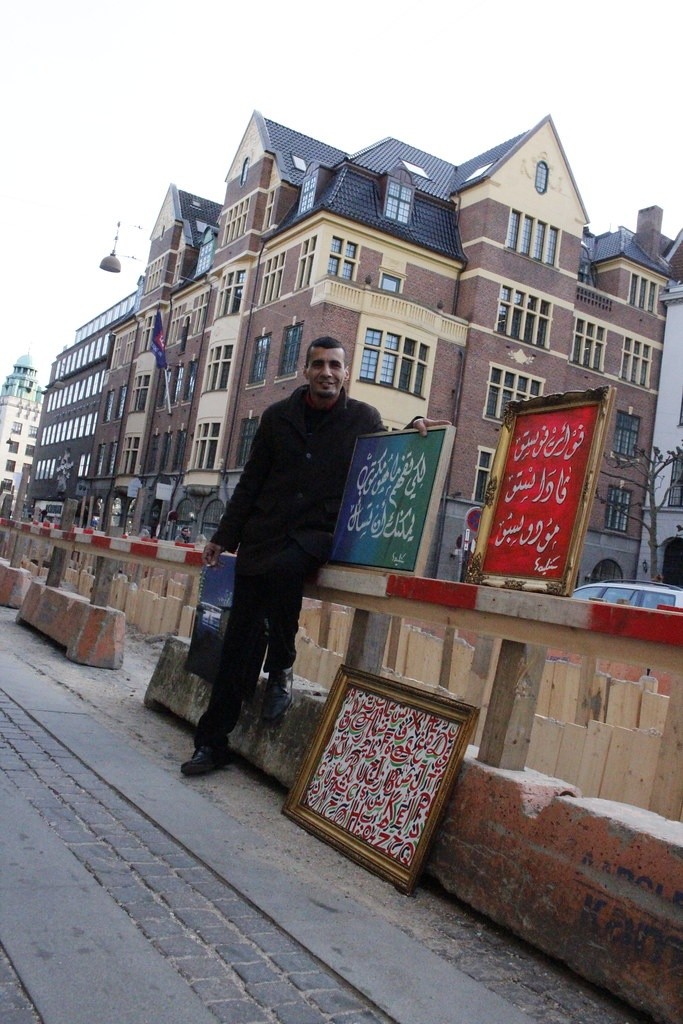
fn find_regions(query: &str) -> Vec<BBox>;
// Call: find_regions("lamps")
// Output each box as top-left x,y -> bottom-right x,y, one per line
99,222 -> 124,274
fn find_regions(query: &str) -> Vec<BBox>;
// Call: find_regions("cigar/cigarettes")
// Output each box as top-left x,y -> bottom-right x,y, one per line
206,564 -> 212,567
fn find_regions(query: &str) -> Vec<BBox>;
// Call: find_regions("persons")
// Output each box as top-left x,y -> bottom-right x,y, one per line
41,509 -> 51,523
175,527 -> 191,544
652,574 -> 663,583
178,336 -> 453,776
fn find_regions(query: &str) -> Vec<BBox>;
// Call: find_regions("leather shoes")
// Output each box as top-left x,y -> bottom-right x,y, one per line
260,665 -> 292,722
182,737 -> 234,776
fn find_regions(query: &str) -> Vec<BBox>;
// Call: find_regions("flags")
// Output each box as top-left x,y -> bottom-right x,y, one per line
149,305 -> 167,371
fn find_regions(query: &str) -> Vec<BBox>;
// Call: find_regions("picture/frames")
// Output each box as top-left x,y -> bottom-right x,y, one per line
462,383 -> 619,598
327,424 -> 458,579
280,663 -> 483,896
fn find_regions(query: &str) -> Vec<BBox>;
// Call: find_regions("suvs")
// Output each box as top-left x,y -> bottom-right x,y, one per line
572,578 -> 682,611
